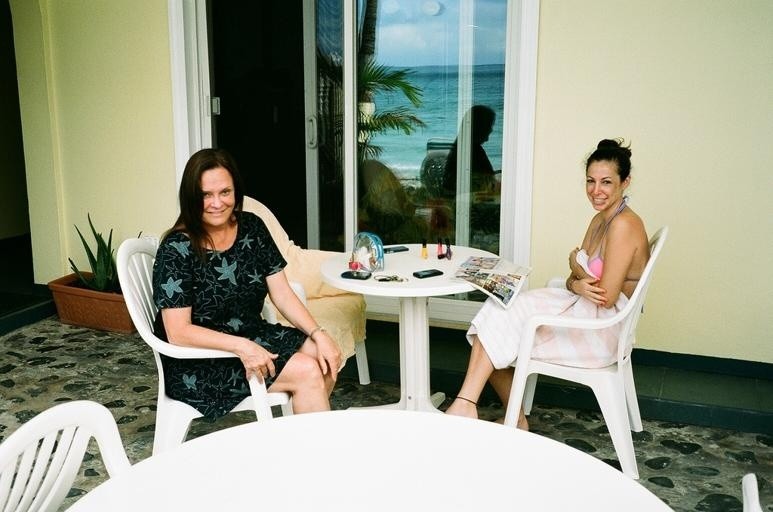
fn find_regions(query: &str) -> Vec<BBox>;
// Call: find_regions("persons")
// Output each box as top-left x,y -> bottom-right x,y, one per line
443,138 -> 651,431
443,105 -> 501,234
151,148 -> 343,419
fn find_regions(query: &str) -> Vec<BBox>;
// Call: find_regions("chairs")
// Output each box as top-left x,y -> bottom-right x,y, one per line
1,398 -> 131,512
503,224 -> 669,479
236,196 -> 371,389
359,152 -> 456,244
118,237 -> 293,454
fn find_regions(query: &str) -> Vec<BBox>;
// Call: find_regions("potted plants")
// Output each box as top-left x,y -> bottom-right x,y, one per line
48,212 -> 143,335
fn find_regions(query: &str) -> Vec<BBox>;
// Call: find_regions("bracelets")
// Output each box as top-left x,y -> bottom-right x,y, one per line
565,277 -> 577,292
310,326 -> 327,341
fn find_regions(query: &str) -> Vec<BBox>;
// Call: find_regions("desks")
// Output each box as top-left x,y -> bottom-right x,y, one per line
67,410 -> 679,512
319,242 -> 500,413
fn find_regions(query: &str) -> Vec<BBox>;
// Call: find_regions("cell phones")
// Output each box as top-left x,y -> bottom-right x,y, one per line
384,246 -> 409,253
415,269 -> 444,278
341,270 -> 371,280
